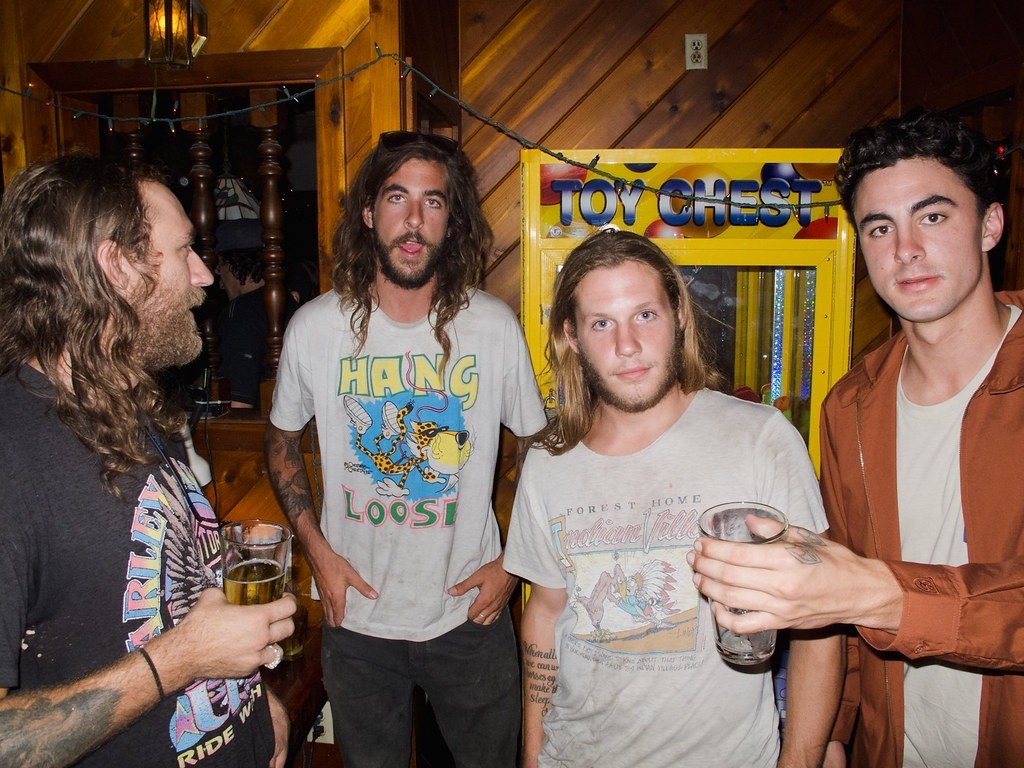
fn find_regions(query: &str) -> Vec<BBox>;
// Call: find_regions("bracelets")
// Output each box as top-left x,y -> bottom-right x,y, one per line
138,647 -> 165,701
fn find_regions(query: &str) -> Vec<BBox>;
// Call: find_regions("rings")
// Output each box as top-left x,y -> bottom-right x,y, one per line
262,644 -> 284,670
479,612 -> 486,620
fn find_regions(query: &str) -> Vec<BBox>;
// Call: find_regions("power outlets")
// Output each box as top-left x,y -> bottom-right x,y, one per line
683,33 -> 708,70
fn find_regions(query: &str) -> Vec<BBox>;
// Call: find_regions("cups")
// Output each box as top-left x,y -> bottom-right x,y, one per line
693,501 -> 790,667
220,519 -> 290,606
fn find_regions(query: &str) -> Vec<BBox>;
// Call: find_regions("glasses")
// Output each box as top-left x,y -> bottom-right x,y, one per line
214,262 -> 227,275
371,130 -> 458,166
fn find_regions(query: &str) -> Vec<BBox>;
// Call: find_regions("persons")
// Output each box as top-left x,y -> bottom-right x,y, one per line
212,243 -> 301,409
502,227 -> 845,768
0,148 -> 292,768
685,103 -> 1024,768
260,122 -> 550,768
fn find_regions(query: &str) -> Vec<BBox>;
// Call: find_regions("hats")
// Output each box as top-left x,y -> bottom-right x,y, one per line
214,220 -> 263,255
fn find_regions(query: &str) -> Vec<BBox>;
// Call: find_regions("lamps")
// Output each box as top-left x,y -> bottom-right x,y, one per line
143,0 -> 209,71
